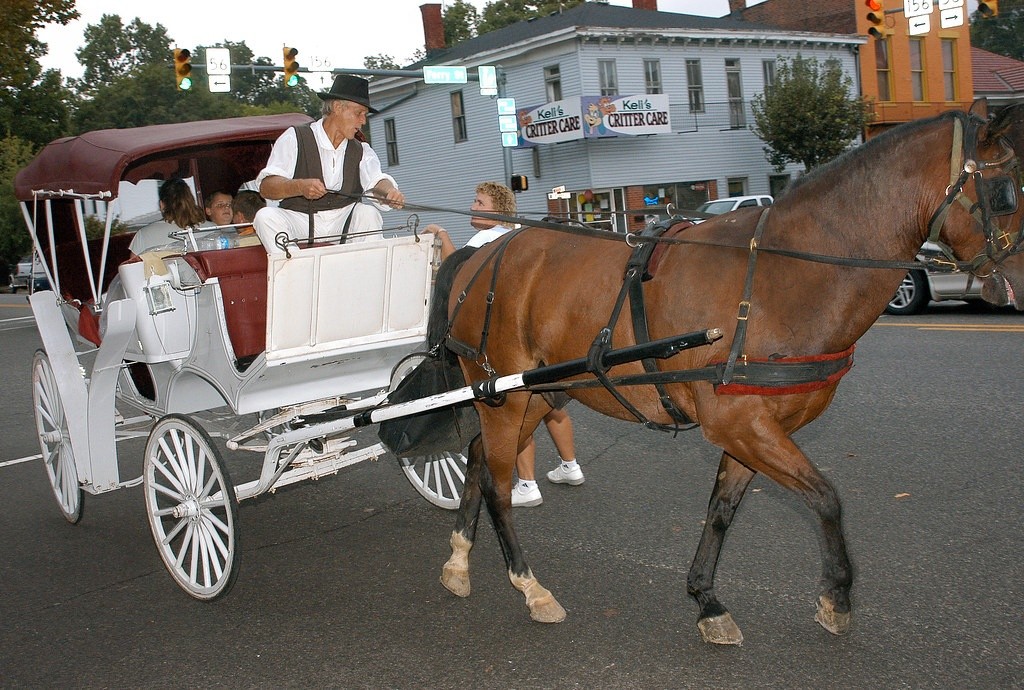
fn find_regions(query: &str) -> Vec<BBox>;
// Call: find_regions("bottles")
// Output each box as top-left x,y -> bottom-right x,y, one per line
186,236 -> 239,250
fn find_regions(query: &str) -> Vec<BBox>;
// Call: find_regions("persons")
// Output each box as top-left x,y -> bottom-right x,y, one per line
230,192 -> 268,241
201,190 -> 234,234
423,181 -> 585,507
130,178 -> 219,259
253,75 -> 405,254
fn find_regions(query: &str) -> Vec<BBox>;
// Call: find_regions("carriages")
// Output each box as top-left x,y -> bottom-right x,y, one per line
18,93 -> 1024,644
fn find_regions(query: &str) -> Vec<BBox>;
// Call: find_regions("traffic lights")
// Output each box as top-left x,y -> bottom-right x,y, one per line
173,48 -> 194,92
282,48 -> 301,89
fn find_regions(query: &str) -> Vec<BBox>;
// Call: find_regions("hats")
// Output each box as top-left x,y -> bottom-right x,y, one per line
317,75 -> 380,114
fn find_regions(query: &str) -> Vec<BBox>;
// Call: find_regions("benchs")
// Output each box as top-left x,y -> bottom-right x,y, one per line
161,221 -> 335,361
41,228 -> 263,363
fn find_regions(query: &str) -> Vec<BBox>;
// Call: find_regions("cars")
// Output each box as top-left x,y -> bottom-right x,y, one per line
28,259 -> 52,292
885,239 -> 983,316
10,263 -> 31,294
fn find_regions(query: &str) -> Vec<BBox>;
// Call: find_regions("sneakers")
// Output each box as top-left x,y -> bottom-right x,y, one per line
511,482 -> 542,507
546,465 -> 585,485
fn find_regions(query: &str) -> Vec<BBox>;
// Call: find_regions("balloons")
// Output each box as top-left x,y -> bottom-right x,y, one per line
584,189 -> 593,202
578,194 -> 586,204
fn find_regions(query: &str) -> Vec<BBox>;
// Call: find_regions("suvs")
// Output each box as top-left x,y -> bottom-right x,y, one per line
688,196 -> 775,228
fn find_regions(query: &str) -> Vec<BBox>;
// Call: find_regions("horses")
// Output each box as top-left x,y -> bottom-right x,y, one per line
426,95 -> 1024,644
